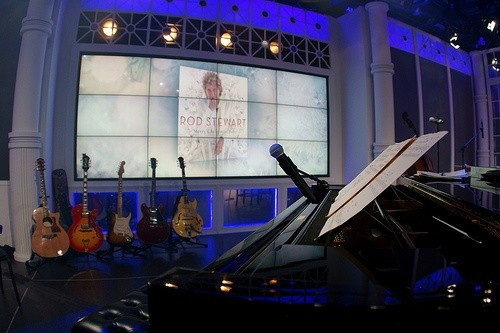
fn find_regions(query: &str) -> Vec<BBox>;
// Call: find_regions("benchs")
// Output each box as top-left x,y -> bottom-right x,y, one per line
71,285 -> 149,333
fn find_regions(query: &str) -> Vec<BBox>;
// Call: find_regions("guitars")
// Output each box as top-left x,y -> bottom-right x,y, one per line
171,157 -> 204,239
137,158 -> 169,244
106,160 -> 134,246
32,158 -> 70,258
68,152 -> 104,252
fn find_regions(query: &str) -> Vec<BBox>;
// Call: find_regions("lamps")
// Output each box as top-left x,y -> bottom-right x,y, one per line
216,33 -> 234,49
163,24 -> 180,43
269,41 -> 282,53
101,17 -> 119,39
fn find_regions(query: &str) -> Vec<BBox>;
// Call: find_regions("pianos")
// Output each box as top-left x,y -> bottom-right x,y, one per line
202,130 -> 500,333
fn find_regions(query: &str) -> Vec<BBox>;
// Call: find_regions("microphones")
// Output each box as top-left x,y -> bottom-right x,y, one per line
429,116 -> 444,124
270,143 -> 318,203
481,122 -> 484,138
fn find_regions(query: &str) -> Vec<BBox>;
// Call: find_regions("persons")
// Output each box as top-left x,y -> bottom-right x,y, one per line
187,72 -> 240,161
